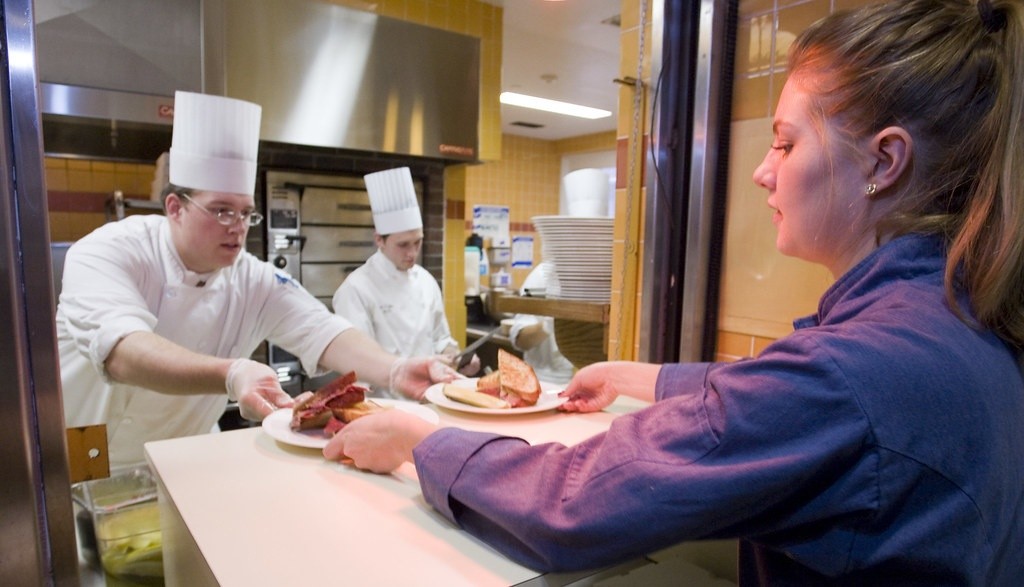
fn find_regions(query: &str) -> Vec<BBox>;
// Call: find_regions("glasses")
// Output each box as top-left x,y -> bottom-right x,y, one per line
182,193 -> 263,228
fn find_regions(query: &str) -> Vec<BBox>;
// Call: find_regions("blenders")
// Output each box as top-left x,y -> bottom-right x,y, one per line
463,246 -> 484,325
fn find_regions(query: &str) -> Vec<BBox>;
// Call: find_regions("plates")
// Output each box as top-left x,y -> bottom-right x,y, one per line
262,398 -> 440,451
527,215 -> 615,304
424,378 -> 570,416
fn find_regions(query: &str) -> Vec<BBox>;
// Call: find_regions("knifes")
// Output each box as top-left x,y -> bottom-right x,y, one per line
453,326 -> 502,361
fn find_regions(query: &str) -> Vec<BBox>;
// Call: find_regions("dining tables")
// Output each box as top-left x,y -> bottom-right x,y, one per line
142,384 -> 658,585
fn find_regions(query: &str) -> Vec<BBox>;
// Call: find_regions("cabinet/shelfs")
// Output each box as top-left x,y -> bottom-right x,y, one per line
213,2 -> 482,163
34,3 -> 211,137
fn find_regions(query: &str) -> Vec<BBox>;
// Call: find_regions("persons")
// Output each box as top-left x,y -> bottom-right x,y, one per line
508,191 -> 624,388
55,127 -> 470,483
460,231 -> 489,305
330,199 -> 481,408
319,0 -> 1024,587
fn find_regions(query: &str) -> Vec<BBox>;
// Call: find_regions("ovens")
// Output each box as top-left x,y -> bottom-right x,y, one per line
264,172 -> 424,395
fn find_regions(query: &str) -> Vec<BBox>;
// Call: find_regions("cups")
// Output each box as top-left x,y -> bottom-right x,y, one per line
500,312 -> 515,336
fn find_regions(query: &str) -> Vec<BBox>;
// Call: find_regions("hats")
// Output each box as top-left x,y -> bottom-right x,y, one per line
170,88 -> 262,195
563,164 -> 613,215
364,165 -> 422,233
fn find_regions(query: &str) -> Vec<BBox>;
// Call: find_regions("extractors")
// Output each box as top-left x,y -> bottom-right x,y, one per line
38,80 -> 176,168
